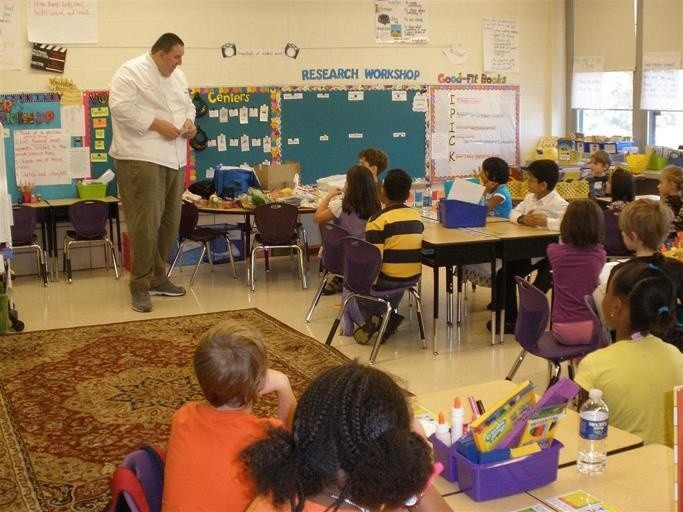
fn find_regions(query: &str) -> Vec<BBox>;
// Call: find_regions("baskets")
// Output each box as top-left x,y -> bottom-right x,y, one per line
498,146 -> 590,200
625,154 -> 651,175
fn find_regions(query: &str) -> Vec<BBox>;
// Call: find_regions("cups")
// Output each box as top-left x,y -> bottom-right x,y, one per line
22,192 -> 31,204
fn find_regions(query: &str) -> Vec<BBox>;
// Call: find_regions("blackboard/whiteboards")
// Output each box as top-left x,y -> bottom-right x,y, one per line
84,85 -> 429,196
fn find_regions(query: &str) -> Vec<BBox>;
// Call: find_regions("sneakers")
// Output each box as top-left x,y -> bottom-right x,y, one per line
149,277 -> 185,296
129,284 -> 152,312
321,276 -> 343,295
487,300 -> 519,333
353,312 -> 404,344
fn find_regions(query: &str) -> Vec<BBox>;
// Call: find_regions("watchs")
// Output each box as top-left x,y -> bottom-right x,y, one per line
400,462 -> 445,512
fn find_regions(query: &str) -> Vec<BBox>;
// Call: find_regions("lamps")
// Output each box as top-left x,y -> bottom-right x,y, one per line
285,43 -> 299,59
220,42 -> 236,58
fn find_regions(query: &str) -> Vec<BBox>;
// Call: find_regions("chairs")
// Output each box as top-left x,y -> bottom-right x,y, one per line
167,204 -> 239,287
4,198 -> 121,287
118,448 -> 165,512
325,236 -> 427,365
305,222 -> 352,323
506,276 -> 610,387
246,204 -> 311,291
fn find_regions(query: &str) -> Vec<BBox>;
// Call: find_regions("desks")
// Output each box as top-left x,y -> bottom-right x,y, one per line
404,378 -> 683,511
409,200 -> 561,355
183,183 -> 447,257
594,194 -> 660,203
520,164 -> 662,180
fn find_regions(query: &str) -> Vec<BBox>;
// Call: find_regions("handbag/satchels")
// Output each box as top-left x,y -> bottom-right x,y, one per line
214,169 -> 260,199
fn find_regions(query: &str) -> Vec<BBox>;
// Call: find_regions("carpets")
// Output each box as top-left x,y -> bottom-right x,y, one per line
1,307 -> 416,511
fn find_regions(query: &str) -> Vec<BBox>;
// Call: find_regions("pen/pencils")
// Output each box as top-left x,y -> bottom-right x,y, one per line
20,182 -> 34,193
417,463 -> 444,503
468,396 -> 485,417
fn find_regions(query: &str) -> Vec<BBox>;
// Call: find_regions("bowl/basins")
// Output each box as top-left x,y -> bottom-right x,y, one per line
626,154 -> 651,172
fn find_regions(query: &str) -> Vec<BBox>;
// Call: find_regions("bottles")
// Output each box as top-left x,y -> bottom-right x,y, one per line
450,396 -> 464,443
577,389 -> 609,477
421,183 -> 433,219
435,412 -> 452,447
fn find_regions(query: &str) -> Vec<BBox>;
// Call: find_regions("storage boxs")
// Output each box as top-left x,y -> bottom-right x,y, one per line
572,139 -> 617,154
214,164 -> 254,198
77,181 -> 107,199
427,424 -> 459,483
451,437 -> 564,502
254,160 -> 301,191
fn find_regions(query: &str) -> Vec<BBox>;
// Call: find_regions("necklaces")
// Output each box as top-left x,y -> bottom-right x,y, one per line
322,489 -> 370,512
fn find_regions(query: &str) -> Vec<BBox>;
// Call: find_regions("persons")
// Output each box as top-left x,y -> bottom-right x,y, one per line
312,148 -> 425,346
586,150 -> 612,198
453,157 -> 512,288
658,164 -> 683,242
603,166 -> 637,257
160,319 -> 298,512
546,198 -> 606,345
602,198 -> 683,354
108,33 -> 197,312
571,262 -> 683,445
486,159 -> 570,334
236,358 -> 456,512
